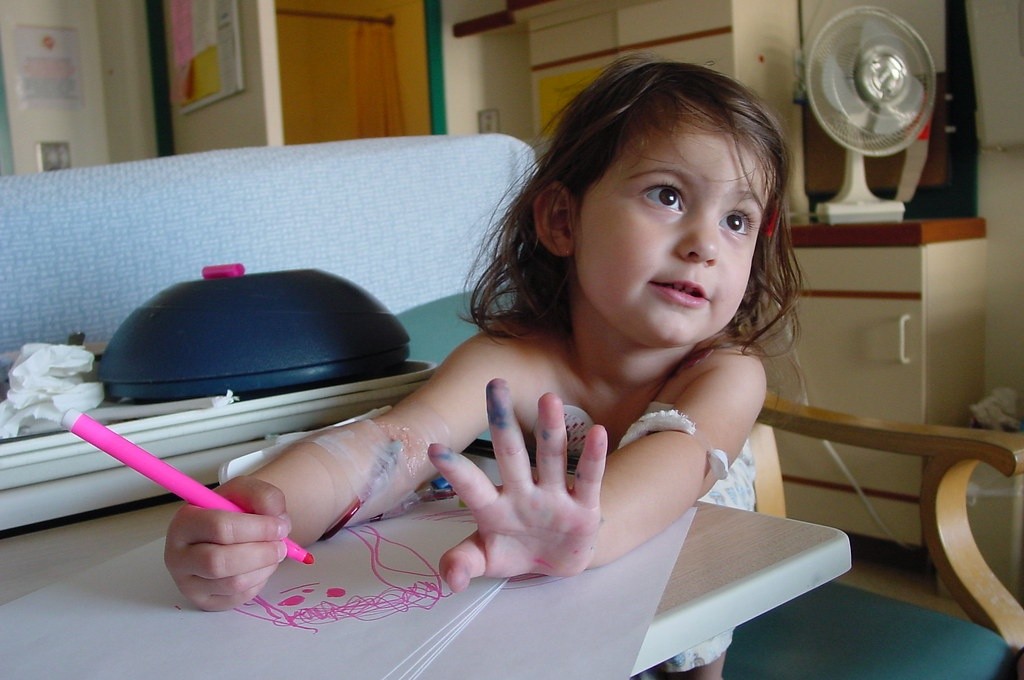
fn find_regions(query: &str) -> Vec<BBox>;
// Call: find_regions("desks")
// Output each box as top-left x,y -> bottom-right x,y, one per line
0,453 -> 853,680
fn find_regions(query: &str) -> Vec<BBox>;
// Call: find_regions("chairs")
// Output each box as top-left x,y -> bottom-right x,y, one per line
398,287 -> 1024,680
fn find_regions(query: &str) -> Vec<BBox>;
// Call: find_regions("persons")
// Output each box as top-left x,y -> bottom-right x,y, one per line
165,51 -> 805,680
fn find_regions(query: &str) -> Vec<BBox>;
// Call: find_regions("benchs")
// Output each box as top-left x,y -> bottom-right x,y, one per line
0,134 -> 539,384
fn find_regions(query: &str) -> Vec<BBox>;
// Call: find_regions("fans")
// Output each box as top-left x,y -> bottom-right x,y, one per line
804,6 -> 936,218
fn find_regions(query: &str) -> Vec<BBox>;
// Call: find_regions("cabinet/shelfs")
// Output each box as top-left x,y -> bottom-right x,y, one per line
452,2 -> 807,222
753,218 -> 990,547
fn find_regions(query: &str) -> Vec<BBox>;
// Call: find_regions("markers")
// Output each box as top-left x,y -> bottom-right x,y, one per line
59,408 -> 315,566
